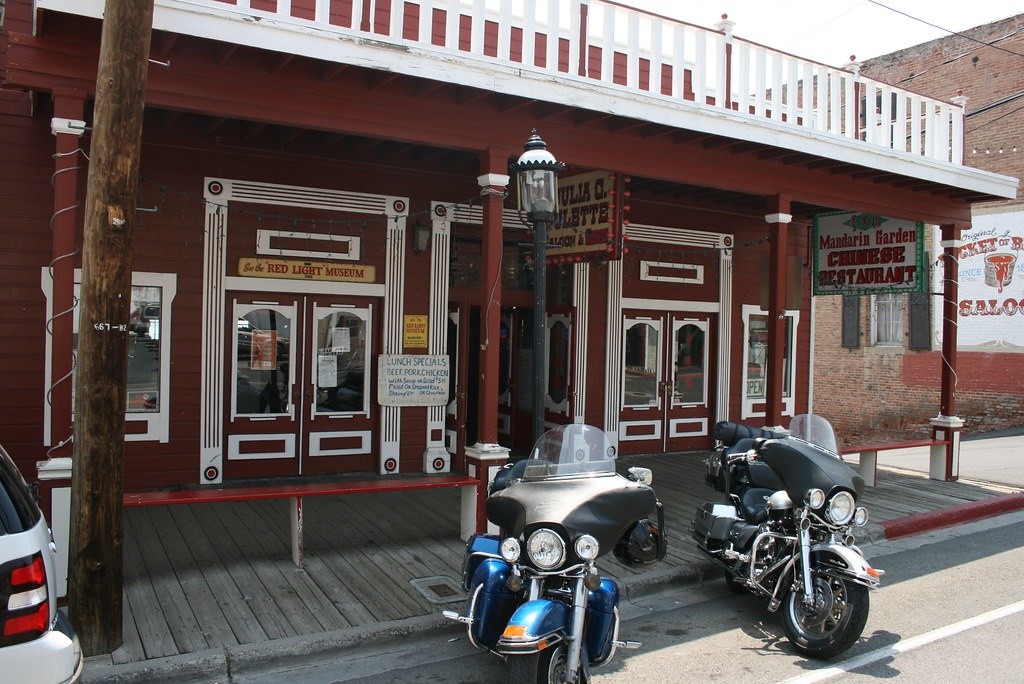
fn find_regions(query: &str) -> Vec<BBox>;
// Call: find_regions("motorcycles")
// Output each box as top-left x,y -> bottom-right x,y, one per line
687,413 -> 885,660
443,422 -> 668,684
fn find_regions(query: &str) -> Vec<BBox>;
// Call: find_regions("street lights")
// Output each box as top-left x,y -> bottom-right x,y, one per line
508,127 -> 567,459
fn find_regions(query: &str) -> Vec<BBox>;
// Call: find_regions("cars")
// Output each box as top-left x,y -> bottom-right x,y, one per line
0,446 -> 83,684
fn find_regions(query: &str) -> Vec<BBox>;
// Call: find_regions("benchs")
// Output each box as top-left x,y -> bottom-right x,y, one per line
122,476 -> 480,569
840,438 -> 949,487
